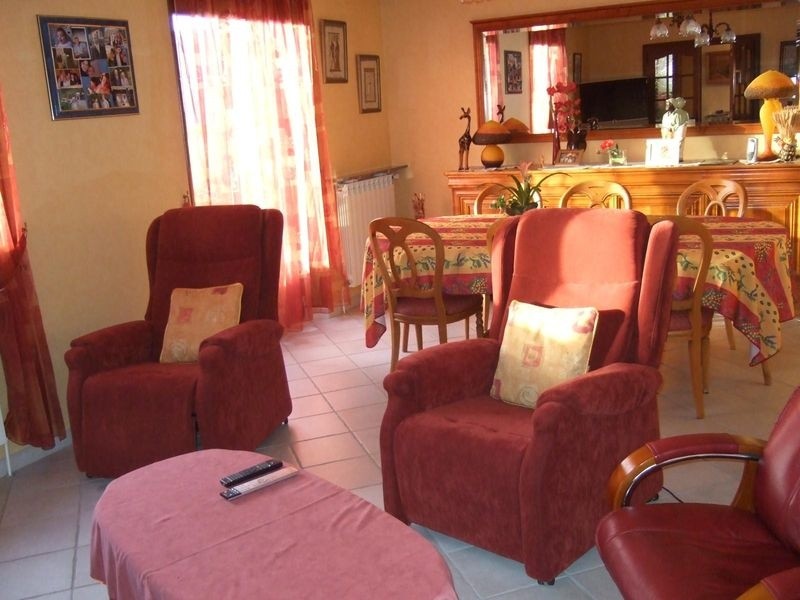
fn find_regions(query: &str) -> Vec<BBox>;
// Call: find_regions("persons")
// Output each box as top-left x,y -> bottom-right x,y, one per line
53,27 -> 130,109
661,97 -> 689,163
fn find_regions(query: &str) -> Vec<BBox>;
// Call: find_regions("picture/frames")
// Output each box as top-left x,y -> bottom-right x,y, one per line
573,52 -> 582,83
555,148 -> 584,166
320,19 -> 349,83
504,50 -> 522,95
356,54 -> 382,113
705,49 -> 730,85
37,15 -> 140,121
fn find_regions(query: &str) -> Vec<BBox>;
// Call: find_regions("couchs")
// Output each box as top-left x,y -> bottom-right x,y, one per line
61,203 -> 292,474
377,203 -> 676,583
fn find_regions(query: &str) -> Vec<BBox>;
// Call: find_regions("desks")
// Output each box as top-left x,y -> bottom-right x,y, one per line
358,215 -> 797,392
86,446 -> 459,600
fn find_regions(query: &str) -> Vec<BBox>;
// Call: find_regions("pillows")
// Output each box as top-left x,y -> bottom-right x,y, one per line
159,282 -> 244,364
489,300 -> 599,410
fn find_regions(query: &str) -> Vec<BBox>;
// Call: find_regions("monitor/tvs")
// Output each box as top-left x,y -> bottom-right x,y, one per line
577,75 -> 654,129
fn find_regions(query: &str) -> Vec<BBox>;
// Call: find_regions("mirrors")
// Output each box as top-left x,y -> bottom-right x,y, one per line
470,1 -> 800,143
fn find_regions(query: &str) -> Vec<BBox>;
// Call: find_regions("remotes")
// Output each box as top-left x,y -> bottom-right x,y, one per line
221,459 -> 284,487
219,467 -> 299,500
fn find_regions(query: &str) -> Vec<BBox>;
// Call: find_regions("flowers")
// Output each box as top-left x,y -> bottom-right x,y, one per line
488,158 -> 570,210
546,86 -> 573,133
556,81 -> 580,128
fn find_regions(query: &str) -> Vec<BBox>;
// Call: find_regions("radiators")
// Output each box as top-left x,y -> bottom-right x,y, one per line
337,171 -> 398,290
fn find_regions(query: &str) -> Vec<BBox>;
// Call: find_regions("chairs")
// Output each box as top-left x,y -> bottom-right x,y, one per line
366,179 -> 746,422
593,384 -> 800,600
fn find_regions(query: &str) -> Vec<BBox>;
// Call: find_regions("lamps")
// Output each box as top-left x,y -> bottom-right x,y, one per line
741,67 -> 792,161
472,120 -> 511,168
647,9 -> 736,47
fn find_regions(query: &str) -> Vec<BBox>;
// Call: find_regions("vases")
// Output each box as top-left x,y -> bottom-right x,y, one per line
505,202 -> 538,217
552,125 -> 560,163
